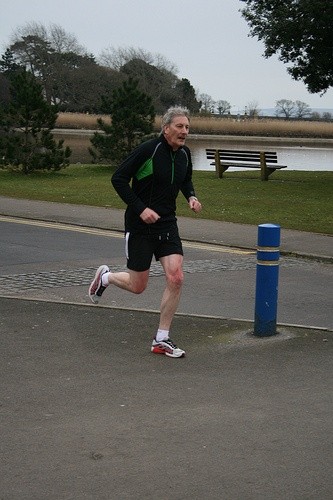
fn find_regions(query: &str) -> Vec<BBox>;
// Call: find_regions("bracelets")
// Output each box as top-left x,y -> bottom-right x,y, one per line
189,196 -> 198,200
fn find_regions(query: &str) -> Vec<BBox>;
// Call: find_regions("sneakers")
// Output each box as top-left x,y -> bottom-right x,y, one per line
88,264 -> 110,304
150,337 -> 185,358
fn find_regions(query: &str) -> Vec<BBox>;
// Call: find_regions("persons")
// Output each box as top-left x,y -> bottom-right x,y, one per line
89,108 -> 201,359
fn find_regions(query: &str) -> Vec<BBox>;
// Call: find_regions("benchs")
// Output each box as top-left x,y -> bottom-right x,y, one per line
205,148 -> 288,182
